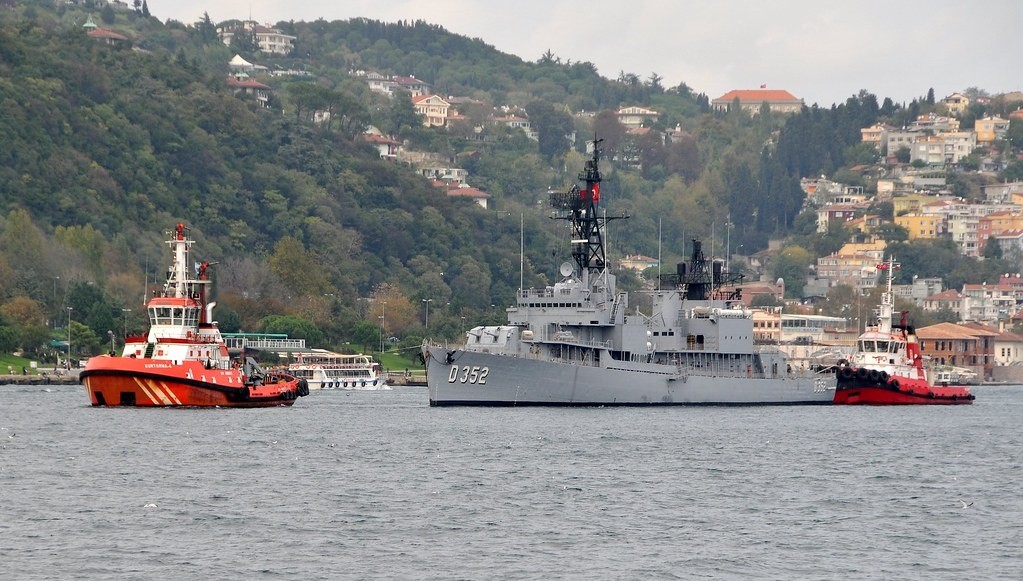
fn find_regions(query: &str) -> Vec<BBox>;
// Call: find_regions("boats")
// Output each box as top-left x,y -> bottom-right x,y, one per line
289,351 -> 387,390
419,130 -> 839,408
78,221 -> 311,409
807,253 -> 978,405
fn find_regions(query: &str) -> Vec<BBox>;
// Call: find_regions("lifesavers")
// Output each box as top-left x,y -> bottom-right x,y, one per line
857,367 -> 868,380
281,379 -> 310,400
362,381 -> 365,387
329,382 -> 333,387
909,388 -> 915,396
336,382 -> 339,387
373,380 -> 377,386
291,364 -> 299,369
344,382 -> 348,387
892,379 -> 900,390
320,365 -> 322,369
968,394 -> 972,400
321,382 -> 325,388
953,395 -> 956,400
306,365 -> 316,369
878,371 -> 888,384
352,381 -> 356,387
841,367 -> 854,380
929,391 -> 936,400
870,369 -> 879,382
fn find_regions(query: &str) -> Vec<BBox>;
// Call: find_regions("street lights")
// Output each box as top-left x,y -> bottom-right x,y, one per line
121,308 -> 132,338
461,315 -> 466,345
856,294 -> 872,338
380,301 -> 388,353
735,245 -> 743,264
422,298 -> 433,329
377,314 -> 384,353
52,276 -> 61,329
67,306 -> 73,370
